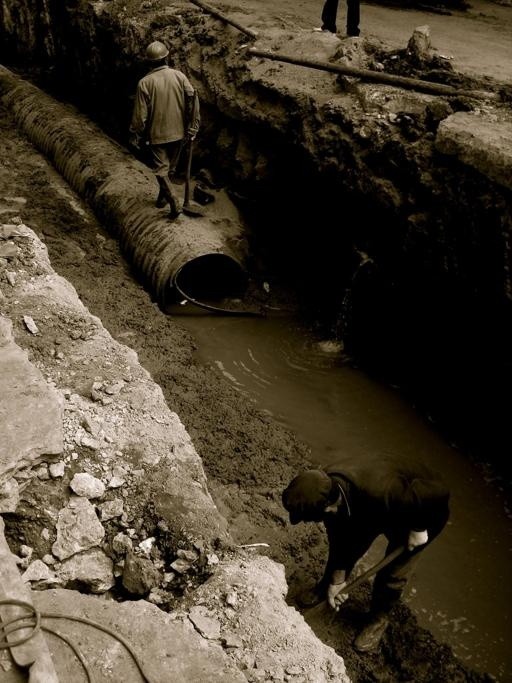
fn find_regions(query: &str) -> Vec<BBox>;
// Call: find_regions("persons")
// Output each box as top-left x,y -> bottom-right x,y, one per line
281,449 -> 450,653
320,0 -> 360,37
127,41 -> 202,219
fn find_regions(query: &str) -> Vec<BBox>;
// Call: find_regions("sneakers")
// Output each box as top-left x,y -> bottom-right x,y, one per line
354,613 -> 389,653
312,26 -> 336,32
296,585 -> 324,609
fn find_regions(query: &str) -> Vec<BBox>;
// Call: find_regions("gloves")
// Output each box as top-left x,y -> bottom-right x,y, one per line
327,581 -> 349,612
186,128 -> 198,141
407,529 -> 428,551
129,135 -> 141,149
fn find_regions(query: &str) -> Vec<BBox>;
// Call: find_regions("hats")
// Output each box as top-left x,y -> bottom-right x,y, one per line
282,470 -> 332,525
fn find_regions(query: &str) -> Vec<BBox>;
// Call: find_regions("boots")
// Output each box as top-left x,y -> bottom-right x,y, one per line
155,174 -> 182,218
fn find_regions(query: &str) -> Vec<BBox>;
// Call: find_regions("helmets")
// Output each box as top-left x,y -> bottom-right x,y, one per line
145,40 -> 170,62
